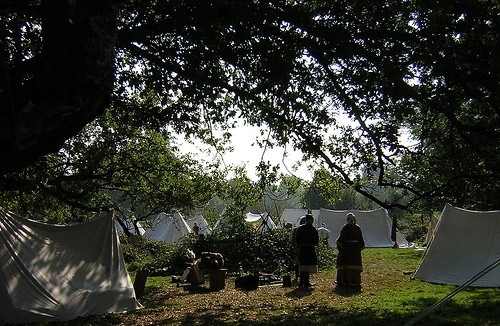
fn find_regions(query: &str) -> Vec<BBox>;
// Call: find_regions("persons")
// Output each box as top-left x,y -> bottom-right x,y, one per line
334,212 -> 364,296
294,215 -> 319,287
290,216 -> 317,286
192,223 -> 199,235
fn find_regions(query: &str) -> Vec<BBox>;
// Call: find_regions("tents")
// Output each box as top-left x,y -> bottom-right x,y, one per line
1,203 -> 500,326
